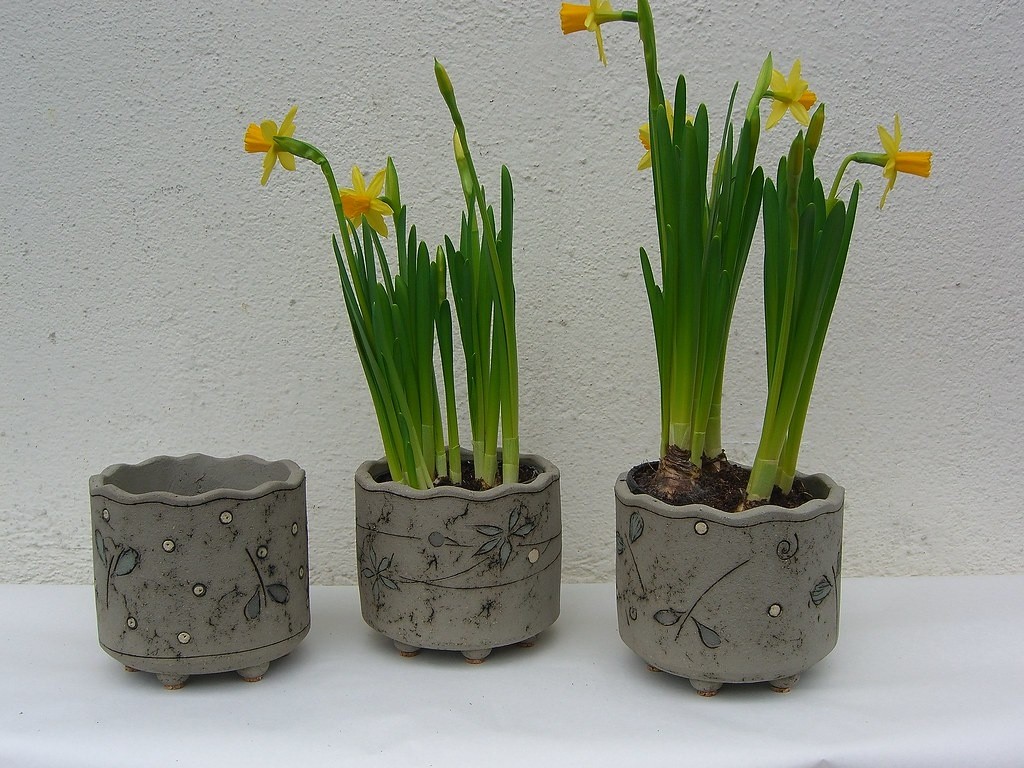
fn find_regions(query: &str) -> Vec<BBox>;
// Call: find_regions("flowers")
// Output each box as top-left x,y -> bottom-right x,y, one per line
558,0 -> 932,499
242,55 -> 520,485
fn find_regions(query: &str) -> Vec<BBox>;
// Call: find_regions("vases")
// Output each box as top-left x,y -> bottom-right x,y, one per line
613,459 -> 846,696
354,445 -> 562,664
89,453 -> 312,689
